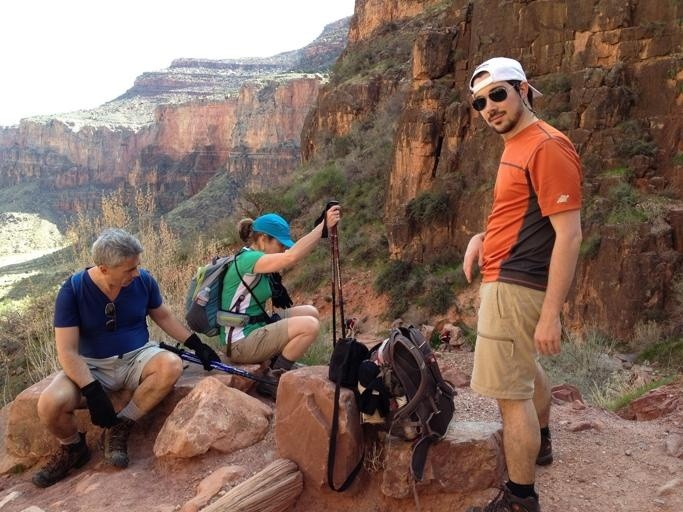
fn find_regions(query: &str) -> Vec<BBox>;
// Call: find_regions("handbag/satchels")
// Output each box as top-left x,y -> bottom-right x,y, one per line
329,338 -> 370,391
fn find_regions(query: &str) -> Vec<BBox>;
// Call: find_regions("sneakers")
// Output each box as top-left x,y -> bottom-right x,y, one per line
257,368 -> 287,399
535,432 -> 553,465
99,411 -> 135,468
31,432 -> 88,487
466,486 -> 540,512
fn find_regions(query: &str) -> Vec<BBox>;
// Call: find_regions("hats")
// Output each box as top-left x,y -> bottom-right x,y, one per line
252,213 -> 295,247
468,57 -> 543,99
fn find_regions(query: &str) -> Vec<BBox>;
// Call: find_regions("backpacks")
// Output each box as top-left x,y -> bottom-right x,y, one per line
185,250 -> 262,336
359,324 -> 454,441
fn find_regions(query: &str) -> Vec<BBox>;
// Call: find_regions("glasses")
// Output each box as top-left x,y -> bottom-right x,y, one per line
105,303 -> 117,330
472,83 -> 518,111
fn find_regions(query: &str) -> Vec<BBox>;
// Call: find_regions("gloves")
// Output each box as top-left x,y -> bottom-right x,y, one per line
183,333 -> 221,372
80,379 -> 118,428
268,272 -> 293,309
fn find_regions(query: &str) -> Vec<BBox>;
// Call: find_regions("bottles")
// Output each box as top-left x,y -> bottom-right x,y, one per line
186,286 -> 210,326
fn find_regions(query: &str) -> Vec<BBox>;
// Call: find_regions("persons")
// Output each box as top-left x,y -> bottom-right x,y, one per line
31,226 -> 226,487
216,202 -> 340,399
464,57 -> 583,512
